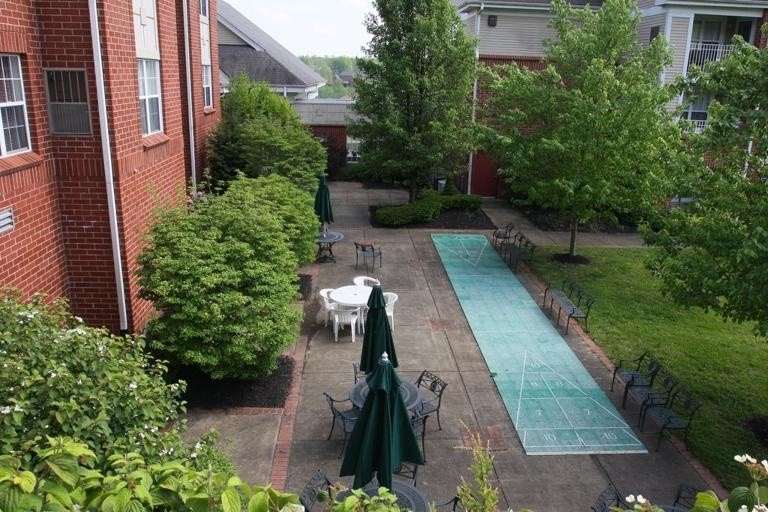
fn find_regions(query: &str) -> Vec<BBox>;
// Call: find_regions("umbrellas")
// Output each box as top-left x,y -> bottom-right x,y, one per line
358,284 -> 400,375
314,173 -> 336,238
336,350 -> 427,497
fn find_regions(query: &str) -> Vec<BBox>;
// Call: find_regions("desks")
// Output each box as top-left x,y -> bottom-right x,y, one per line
314,231 -> 344,264
333,476 -> 430,512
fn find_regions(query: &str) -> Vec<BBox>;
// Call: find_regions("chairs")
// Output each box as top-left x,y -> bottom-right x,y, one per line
297,470 -> 334,511
322,360 -> 448,484
492,221 -> 537,275
353,240 -> 383,273
543,275 -> 595,336
318,276 -> 398,343
434,491 -> 477,512
672,484 -> 703,510
591,483 -> 633,511
610,350 -> 704,453
